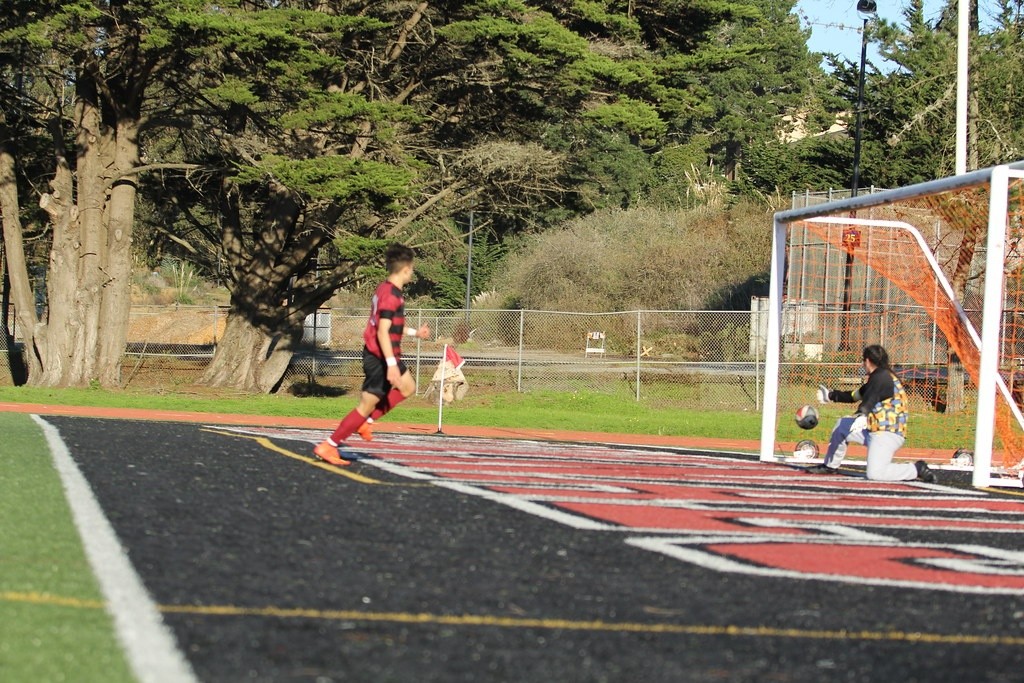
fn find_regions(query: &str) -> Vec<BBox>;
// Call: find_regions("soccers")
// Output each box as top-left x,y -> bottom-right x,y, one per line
795,405 -> 819,429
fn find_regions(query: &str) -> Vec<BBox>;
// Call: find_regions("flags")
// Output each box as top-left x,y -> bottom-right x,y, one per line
443,343 -> 466,371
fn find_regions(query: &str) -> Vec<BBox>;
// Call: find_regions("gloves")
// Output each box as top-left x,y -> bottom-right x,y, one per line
817,384 -> 831,405
849,414 -> 867,433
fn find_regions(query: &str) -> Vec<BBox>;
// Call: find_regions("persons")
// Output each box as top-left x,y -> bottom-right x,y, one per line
313,242 -> 430,467
804,344 -> 939,483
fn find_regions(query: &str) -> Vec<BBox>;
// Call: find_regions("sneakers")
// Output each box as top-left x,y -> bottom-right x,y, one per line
358,420 -> 373,442
915,461 -> 937,483
804,464 -> 838,475
313,440 -> 352,466
